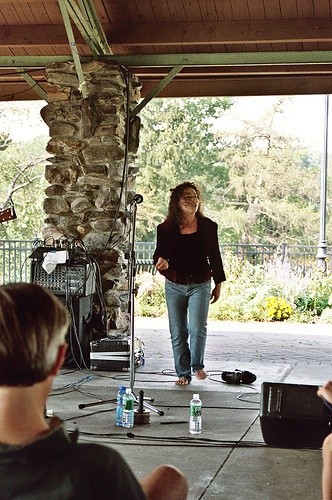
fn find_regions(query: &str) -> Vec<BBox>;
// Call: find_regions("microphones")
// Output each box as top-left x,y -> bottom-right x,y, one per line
130,194 -> 143,206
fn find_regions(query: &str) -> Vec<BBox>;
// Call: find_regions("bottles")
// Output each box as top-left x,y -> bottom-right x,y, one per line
123,389 -> 134,427
188,394 -> 202,435
116,386 -> 125,426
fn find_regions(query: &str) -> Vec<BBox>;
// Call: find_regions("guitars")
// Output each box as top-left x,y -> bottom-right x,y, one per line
0,207 -> 17,224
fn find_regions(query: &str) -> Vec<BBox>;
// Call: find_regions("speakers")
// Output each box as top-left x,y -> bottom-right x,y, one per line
54,294 -> 93,370
260,382 -> 332,450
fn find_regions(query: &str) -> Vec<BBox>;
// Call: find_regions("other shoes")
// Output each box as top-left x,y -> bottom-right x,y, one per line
222,371 -> 242,384
235,370 -> 256,383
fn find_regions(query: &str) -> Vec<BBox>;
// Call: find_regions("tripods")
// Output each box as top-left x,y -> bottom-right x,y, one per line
78,207 -> 165,417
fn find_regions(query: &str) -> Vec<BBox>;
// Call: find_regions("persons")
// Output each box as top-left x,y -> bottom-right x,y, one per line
153,182 -> 226,385
317,381 -> 332,500
0,282 -> 188,500
